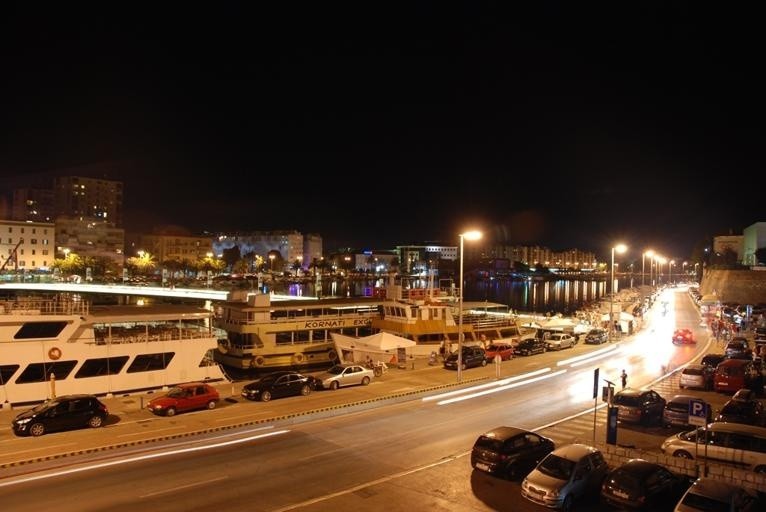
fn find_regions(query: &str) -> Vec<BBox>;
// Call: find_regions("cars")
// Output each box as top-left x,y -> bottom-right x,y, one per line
673,476 -> 757,512
239,370 -> 317,402
662,394 -> 713,428
146,382 -> 221,417
520,443 -> 610,512
612,386 -> 667,427
470,426 -> 556,481
314,363 -> 375,391
444,327 -> 608,371
11,393 -> 109,437
597,458 -> 678,512
671,326 -> 766,424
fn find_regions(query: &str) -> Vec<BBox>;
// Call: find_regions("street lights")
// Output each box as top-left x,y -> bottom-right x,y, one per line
641,249 -> 699,306
457,230 -> 482,382
609,244 -> 627,343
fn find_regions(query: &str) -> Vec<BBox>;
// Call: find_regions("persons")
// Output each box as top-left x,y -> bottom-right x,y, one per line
620,369 -> 627,390
388,355 -> 396,368
366,355 -> 374,369
430,351 -> 436,363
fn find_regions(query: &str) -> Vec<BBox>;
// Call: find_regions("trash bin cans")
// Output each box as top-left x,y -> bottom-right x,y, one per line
440,347 -> 445,354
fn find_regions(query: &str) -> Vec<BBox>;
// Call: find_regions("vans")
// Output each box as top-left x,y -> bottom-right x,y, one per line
660,420 -> 766,474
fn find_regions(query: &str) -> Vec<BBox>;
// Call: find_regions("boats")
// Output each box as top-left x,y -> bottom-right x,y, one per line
330,294 -> 528,365
214,295 -> 382,375
0,294 -> 234,411
